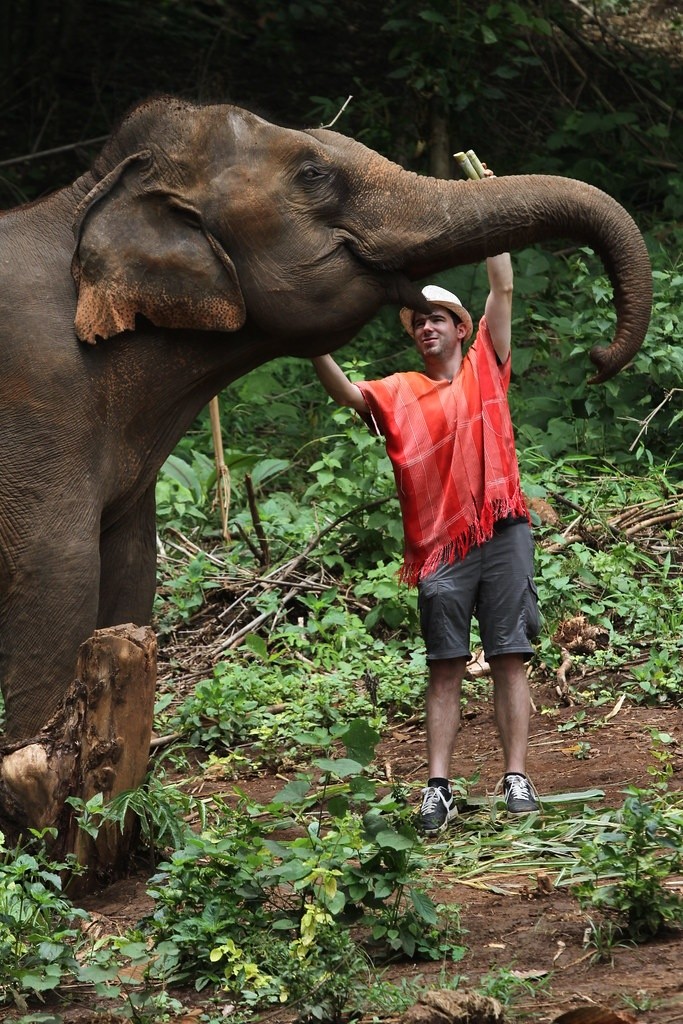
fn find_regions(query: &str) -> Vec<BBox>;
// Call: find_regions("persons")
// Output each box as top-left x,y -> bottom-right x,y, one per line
308,164 -> 543,835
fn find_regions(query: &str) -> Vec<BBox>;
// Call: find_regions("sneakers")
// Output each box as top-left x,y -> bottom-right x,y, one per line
418,784 -> 458,835
502,774 -> 539,818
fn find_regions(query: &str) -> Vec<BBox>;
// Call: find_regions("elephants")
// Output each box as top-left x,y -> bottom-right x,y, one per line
0,91 -> 655,893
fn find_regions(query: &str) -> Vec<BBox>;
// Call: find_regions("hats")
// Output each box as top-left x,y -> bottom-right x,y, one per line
398,285 -> 473,342
